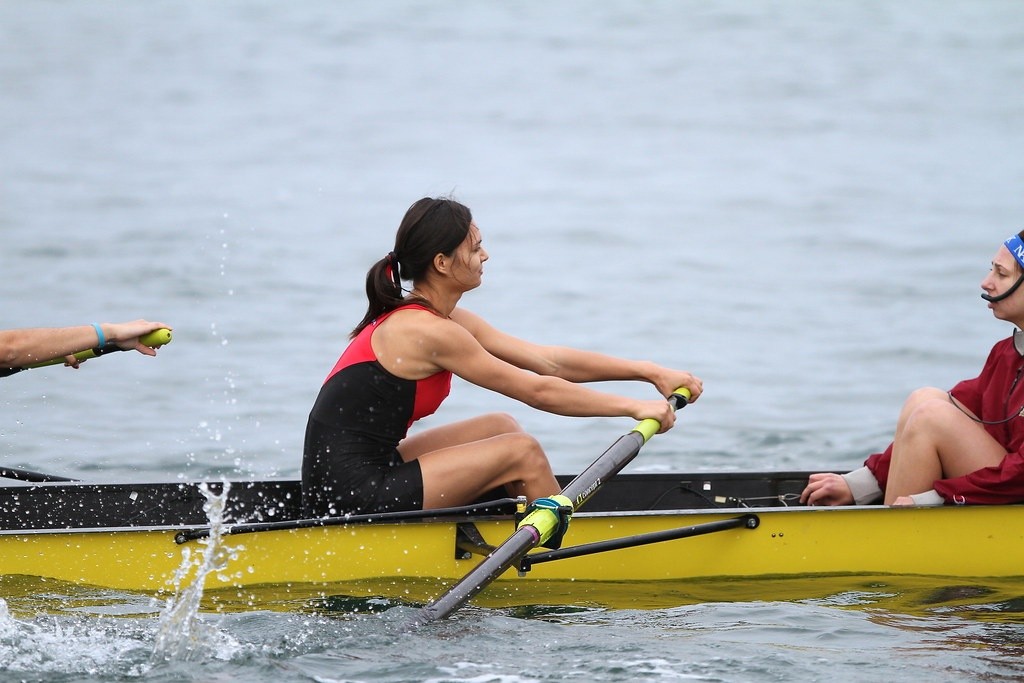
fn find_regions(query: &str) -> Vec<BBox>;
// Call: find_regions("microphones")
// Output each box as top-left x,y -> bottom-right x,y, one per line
980,273 -> 1024,301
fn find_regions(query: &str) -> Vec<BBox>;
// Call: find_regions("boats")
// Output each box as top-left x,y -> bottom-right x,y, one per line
0,469 -> 1024,627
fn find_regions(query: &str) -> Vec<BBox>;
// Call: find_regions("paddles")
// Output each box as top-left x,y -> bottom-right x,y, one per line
0,324 -> 174,390
420,377 -> 702,620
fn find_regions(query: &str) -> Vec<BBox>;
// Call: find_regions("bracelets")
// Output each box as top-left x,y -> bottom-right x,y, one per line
91,323 -> 106,348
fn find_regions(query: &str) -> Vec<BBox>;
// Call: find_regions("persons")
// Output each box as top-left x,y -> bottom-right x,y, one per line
0,319 -> 172,369
301,195 -> 703,519
799,231 -> 1024,505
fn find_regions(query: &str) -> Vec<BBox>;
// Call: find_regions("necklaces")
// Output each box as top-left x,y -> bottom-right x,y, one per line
414,289 -> 428,300
